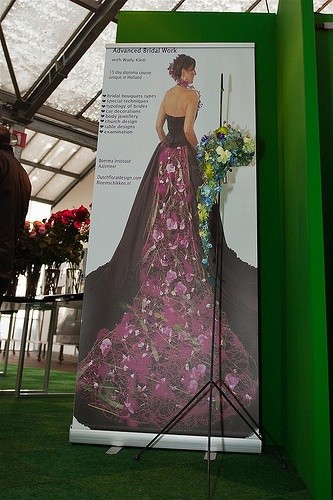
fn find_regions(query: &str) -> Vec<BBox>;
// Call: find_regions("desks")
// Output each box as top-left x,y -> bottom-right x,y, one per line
1,293 -> 84,400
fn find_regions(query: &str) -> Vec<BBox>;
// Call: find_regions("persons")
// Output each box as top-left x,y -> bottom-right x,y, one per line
0,123 -> 31,309
74,53 -> 259,440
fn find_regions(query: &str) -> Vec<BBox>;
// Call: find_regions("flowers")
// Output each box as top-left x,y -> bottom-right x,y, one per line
18,203 -> 91,274
194,124 -> 255,266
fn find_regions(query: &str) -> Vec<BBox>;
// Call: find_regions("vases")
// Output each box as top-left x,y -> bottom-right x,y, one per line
42,267 -> 61,295
65,267 -> 83,295
24,270 -> 41,300
